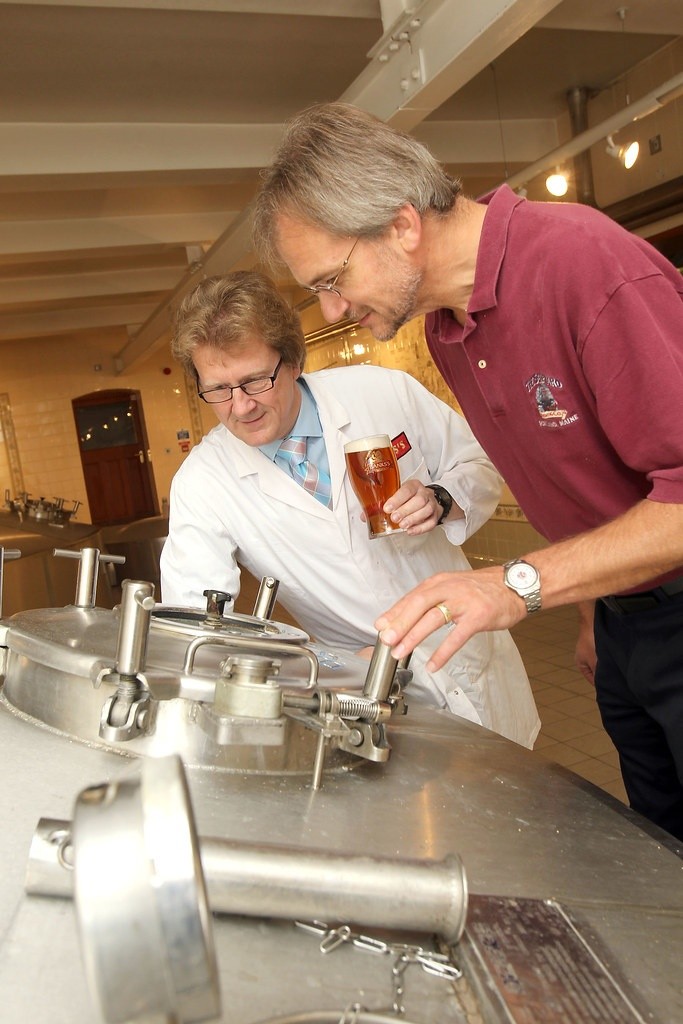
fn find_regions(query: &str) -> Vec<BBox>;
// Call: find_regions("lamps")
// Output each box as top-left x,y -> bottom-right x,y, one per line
546,168 -> 571,197
603,133 -> 639,169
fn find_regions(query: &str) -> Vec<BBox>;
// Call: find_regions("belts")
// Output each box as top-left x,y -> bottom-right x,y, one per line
600,582 -> 682,616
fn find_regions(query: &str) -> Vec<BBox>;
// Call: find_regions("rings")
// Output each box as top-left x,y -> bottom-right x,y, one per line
437,604 -> 452,624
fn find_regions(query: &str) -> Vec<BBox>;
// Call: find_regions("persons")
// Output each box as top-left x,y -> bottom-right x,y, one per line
161,271 -> 540,751
254,101 -> 683,841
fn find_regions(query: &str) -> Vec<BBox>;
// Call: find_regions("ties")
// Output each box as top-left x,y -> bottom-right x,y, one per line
276,436 -> 333,512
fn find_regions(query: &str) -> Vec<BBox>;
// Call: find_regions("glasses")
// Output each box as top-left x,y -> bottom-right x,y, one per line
197,357 -> 283,404
303,236 -> 360,300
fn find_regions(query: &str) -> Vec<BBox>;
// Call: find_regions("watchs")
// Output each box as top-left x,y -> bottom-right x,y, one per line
427,485 -> 453,526
504,559 -> 542,614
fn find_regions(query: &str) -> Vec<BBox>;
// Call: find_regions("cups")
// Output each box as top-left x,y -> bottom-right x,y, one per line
344,435 -> 408,538
